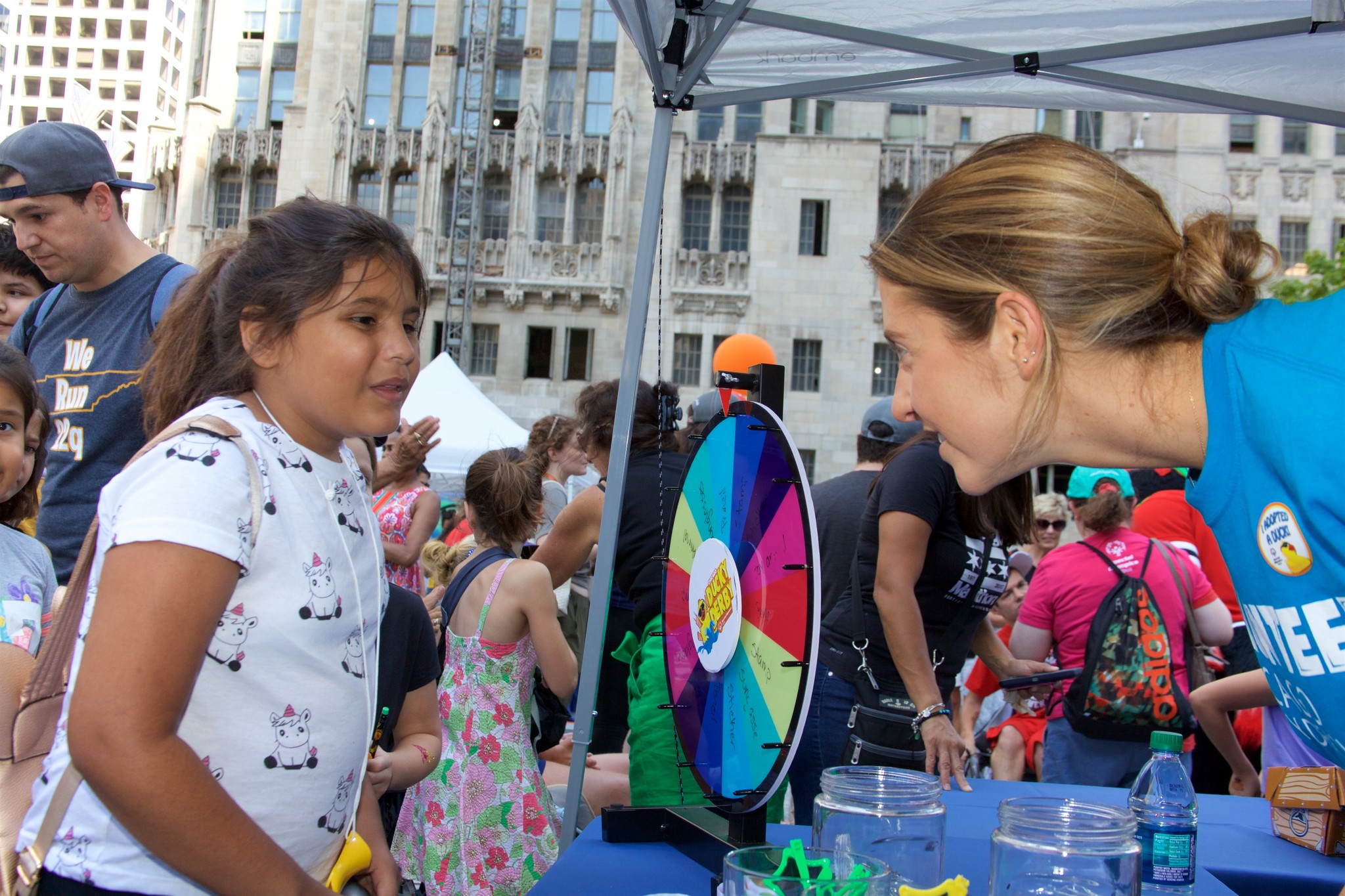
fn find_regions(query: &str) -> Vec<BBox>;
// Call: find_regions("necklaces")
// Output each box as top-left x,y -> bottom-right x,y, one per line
1182,341 -> 1216,490
250,385 -> 387,892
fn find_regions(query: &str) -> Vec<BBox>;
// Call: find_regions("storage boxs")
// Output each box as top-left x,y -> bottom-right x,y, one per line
1265,766 -> 1345,855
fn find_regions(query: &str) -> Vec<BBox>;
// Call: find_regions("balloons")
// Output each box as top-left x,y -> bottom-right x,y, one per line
712,332 -> 776,396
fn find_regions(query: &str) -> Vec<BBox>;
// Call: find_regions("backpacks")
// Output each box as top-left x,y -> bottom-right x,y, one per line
1062,538 -> 1201,744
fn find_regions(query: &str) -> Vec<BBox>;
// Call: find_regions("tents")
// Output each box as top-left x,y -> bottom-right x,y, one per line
376,349 -> 602,503
561,0 -> 1344,865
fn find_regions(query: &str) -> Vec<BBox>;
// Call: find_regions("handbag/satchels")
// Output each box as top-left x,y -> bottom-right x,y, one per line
0,410 -> 265,896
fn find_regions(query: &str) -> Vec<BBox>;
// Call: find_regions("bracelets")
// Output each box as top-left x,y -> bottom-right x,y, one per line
914,710 -> 950,731
909,701 -> 945,730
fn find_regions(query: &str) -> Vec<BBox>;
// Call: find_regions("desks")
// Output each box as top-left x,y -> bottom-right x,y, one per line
531,768 -> 1345,896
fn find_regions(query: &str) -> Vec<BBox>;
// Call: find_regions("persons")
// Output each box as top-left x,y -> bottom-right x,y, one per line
20,202 -> 421,895
0,120 -> 213,588
0,226 -> 59,658
340,378 -> 749,896
871,132 -> 1345,789
790,393 -> 1345,825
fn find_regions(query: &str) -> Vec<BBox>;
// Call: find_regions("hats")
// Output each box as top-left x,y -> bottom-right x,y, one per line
1006,551 -> 1034,578
1066,465 -> 1136,501
860,395 -> 924,444
684,393 -> 740,427
0,120 -> 158,202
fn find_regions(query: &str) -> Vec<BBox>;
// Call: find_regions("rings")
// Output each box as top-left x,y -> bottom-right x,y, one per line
434,617 -> 438,626
414,430 -> 420,439
418,440 -> 425,446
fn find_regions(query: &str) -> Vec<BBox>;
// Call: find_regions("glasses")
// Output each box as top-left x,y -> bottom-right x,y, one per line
1033,518 -> 1067,532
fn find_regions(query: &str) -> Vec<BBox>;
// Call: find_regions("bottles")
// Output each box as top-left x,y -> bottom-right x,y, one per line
1127,730 -> 1199,896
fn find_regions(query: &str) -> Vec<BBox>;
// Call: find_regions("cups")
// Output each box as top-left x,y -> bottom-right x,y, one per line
809,765 -> 947,896
988,796 -> 1142,896
722,844 -> 892,896
